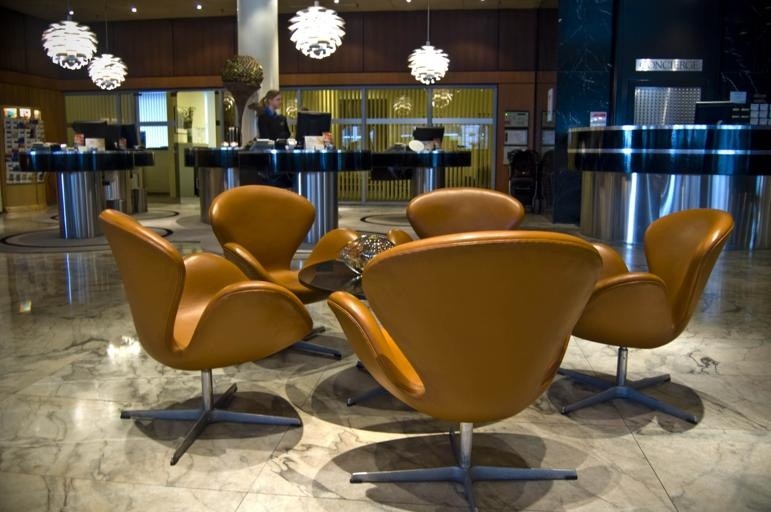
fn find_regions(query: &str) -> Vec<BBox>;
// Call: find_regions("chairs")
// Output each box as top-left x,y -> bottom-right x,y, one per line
327,229 -> 604,512
208,184 -> 361,365
388,186 -> 527,247
558,208 -> 736,427
99,206 -> 314,468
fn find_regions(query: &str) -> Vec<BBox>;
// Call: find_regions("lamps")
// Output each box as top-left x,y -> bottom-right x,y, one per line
287,0 -> 346,60
407,0 -> 450,86
86,0 -> 128,91
41,0 -> 99,71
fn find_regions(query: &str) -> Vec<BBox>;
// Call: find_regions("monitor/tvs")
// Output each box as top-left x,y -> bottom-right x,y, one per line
457,124 -> 488,150
294,112 -> 330,149
107,124 -> 138,150
342,124 -> 377,151
415,128 -> 444,142
695,102 -> 733,125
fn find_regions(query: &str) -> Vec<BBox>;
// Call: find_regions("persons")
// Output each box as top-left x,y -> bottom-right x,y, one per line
256,90 -> 291,149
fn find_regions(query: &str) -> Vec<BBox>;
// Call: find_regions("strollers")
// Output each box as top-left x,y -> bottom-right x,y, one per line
503,148 -> 541,215
